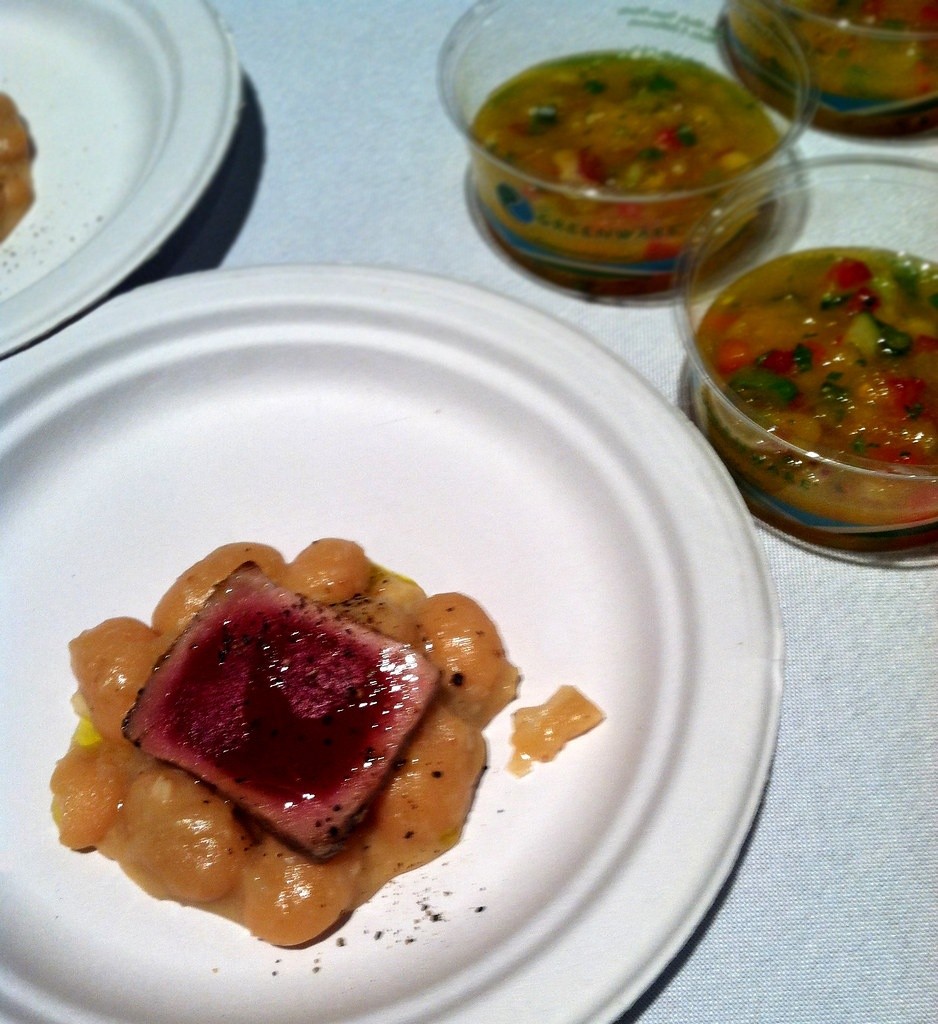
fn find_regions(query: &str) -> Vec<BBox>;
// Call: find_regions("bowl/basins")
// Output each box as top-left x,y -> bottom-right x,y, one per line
434,0 -> 822,302
668,150 -> 938,552
713,0 -> 938,143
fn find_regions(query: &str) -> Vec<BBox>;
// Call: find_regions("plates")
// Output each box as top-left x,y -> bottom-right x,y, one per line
0,260 -> 786,1023
0,0 -> 252,360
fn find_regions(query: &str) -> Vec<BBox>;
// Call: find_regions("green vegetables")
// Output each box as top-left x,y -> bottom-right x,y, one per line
525,73 -> 700,169
727,261 -> 938,491
